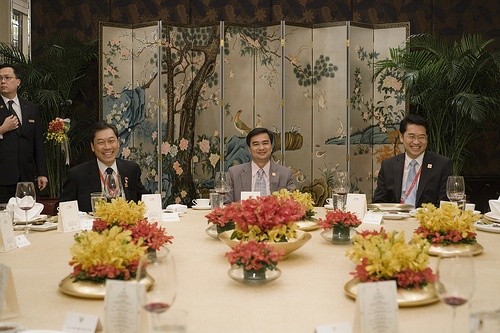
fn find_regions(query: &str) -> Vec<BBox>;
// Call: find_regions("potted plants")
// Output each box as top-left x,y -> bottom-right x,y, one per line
0,35 -> 92,213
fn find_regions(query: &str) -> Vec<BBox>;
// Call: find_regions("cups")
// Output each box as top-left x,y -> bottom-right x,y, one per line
334,171 -> 351,195
326,198 -> 333,205
445,175 -> 465,202
210,192 -> 220,211
90,192 -> 107,213
332,194 -> 344,212
465,203 -> 475,211
488,199 -> 500,218
192,199 -> 209,207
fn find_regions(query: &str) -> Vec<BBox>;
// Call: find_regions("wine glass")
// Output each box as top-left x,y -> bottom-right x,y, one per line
137,246 -> 179,333
434,247 -> 475,333
213,171 -> 230,209
15,182 -> 37,228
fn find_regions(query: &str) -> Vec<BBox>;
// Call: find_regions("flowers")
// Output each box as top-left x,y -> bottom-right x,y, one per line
70,199 -> 173,276
45,116 -> 71,166
206,189 -> 477,284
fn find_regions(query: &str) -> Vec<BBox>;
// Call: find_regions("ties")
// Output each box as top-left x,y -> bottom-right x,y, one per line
105,168 -> 116,203
254,169 -> 266,197
7,100 -> 21,128
405,160 -> 418,203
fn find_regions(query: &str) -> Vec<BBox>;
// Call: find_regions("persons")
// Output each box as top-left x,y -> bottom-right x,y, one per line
55,121 -> 152,222
219,128 -> 295,209
371,115 -> 455,210
0,63 -> 49,213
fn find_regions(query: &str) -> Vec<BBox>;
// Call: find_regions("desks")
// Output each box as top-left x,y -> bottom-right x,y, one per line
0,196 -> 499,333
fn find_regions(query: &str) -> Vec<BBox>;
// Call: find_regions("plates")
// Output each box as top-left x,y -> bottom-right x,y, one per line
484,212 -> 500,224
14,221 -> 59,231
473,221 -> 500,234
409,238 -> 484,259
296,218 -> 323,231
324,205 -> 333,210
58,271 -> 154,300
206,224 -> 219,239
191,206 -> 212,210
369,203 -> 414,212
342,276 -> 448,307
472,210 -> 481,214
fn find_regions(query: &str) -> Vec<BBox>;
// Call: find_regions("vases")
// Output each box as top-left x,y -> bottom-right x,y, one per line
216,228 -> 312,259
344,280 -> 448,307
319,226 -> 359,245
295,218 -> 322,230
407,241 -> 484,258
60,272 -> 154,299
228,262 -> 280,286
206,223 -> 238,239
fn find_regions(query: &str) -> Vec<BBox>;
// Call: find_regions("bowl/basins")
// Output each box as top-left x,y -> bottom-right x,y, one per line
218,227 -> 312,257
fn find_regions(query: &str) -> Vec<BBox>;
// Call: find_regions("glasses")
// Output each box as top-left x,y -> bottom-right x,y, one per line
0,76 -> 16,81
404,134 -> 427,141
